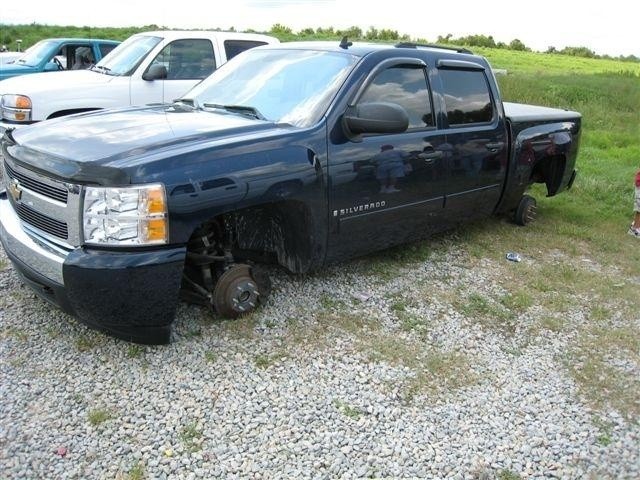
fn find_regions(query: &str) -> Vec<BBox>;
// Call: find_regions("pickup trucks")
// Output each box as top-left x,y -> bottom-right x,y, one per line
0,35 -> 585,346
0,29 -> 283,145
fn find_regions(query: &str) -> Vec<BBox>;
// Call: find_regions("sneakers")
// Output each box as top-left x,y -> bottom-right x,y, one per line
627,227 -> 640,239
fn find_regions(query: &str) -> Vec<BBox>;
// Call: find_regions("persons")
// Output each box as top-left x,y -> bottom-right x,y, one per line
449,134 -> 503,178
532,132 -> 571,197
626,168 -> 640,239
369,144 -> 412,194
418,145 -> 443,182
516,138 -> 535,187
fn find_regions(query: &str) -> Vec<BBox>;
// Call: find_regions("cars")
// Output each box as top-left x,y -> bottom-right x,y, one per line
0,38 -> 123,83
